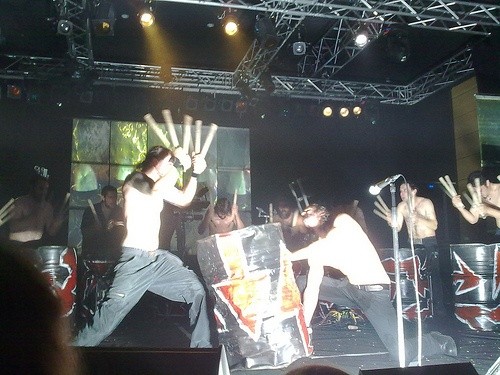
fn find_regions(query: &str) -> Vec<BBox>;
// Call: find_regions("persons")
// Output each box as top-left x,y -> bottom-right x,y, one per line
158,180 -> 206,251
386,181 -> 445,320
81,184 -> 123,260
273,198 -> 369,273
452,170 -> 500,243
68,143 -> 214,349
6,174 -> 66,242
198,199 -> 244,237
0,241 -> 79,375
279,199 -> 457,364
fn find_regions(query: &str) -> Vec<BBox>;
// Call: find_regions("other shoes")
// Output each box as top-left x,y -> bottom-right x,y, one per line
428,330 -> 457,356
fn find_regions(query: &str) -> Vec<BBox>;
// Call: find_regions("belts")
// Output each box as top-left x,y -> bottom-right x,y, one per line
355,284 -> 390,291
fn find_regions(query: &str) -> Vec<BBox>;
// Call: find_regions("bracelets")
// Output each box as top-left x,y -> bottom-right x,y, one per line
190,172 -> 199,178
169,156 -> 184,175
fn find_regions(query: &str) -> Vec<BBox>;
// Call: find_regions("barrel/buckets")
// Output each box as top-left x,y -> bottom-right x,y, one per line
449,242 -> 500,332
377,248 -> 431,336
32,246 -> 77,344
198,222 -> 314,373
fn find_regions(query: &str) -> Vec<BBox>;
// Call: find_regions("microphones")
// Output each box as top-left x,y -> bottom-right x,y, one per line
369,174 -> 401,195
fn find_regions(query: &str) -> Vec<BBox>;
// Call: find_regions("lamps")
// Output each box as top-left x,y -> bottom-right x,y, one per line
92,3 -> 116,36
255,14 -> 279,51
56,19 -> 73,37
287,38 -> 306,56
387,35 -> 408,63
